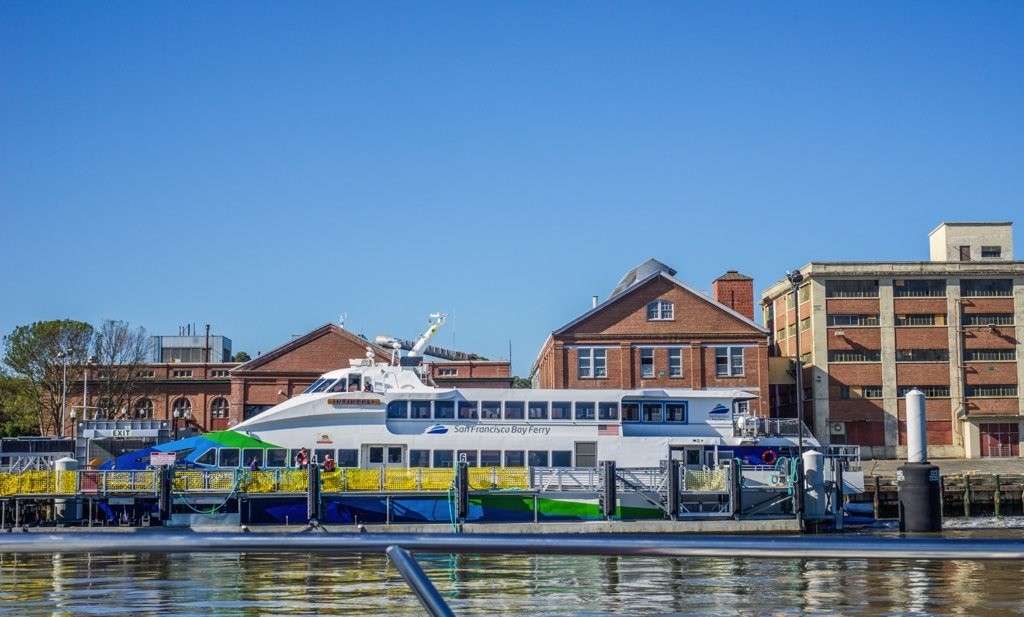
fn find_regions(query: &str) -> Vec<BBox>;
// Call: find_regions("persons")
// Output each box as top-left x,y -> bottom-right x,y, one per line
365,381 -> 373,392
323,455 -> 335,472
296,447 -> 309,469
251,456 -> 260,471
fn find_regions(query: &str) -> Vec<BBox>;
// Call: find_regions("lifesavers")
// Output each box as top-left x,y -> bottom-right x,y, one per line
88,459 -> 103,470
323,458 -> 335,470
762,450 -> 778,465
295,451 -> 310,466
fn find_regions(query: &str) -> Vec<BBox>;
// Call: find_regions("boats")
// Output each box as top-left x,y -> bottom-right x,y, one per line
93,310 -> 867,524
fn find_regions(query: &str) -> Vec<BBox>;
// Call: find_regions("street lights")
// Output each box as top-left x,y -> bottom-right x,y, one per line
69,408 -> 76,457
173,407 -> 180,442
